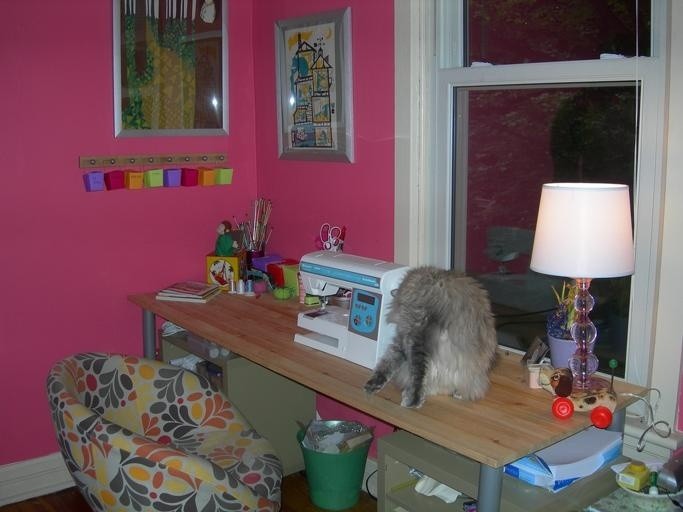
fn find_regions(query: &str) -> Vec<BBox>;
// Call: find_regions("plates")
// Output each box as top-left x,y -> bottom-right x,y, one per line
616,472 -> 682,501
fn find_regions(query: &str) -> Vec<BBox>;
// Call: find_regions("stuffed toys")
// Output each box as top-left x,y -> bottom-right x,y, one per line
214,221 -> 238,257
539,368 -> 617,428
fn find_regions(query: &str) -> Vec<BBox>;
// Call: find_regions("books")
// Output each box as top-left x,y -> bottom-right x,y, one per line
155,281 -> 222,304
503,426 -> 623,492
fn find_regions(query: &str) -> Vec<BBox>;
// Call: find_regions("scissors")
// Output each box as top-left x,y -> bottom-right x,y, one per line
320,223 -> 342,251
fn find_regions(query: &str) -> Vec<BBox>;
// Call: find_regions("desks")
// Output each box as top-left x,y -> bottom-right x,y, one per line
126,283 -> 649,511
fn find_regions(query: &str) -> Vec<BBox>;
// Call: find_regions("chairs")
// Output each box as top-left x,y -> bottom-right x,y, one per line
45,352 -> 285,511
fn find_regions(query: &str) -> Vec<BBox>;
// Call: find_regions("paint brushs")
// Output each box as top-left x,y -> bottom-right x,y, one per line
233,198 -> 274,252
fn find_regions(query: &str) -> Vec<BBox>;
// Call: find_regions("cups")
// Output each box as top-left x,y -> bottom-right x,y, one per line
548,335 -> 577,369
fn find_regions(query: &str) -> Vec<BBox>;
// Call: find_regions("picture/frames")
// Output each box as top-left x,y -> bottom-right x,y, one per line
273,6 -> 356,163
112,0 -> 229,136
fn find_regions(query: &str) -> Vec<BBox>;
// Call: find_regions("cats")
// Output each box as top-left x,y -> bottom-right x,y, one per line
364,265 -> 498,409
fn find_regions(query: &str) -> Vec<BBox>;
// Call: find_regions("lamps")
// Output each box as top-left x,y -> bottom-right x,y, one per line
529,183 -> 636,374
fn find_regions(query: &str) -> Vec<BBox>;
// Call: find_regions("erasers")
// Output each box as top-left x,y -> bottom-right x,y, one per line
631,460 -> 645,472
